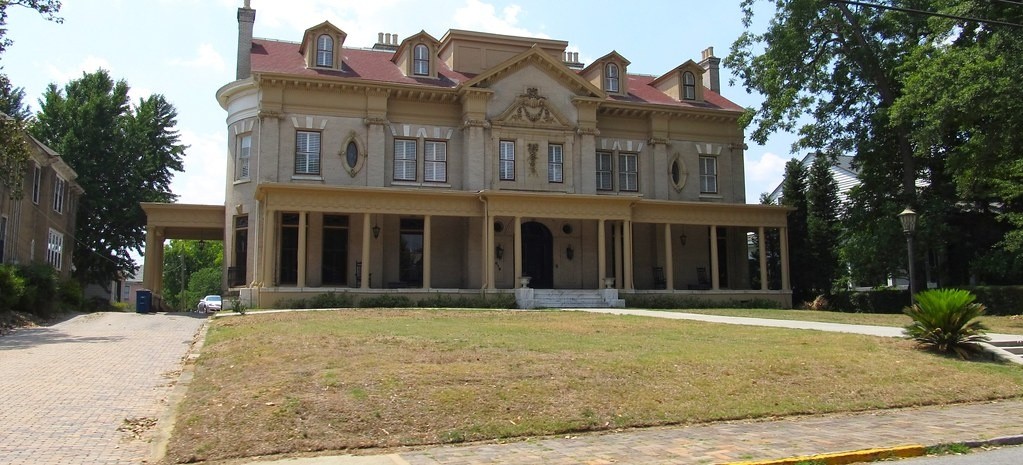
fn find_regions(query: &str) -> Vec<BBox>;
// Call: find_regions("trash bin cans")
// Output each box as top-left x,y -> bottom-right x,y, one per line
135,288 -> 153,313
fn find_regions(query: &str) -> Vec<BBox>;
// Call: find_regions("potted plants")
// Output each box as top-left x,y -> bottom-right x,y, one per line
518,276 -> 532,288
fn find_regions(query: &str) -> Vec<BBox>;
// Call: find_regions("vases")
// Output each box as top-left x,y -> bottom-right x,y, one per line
603,277 -> 616,290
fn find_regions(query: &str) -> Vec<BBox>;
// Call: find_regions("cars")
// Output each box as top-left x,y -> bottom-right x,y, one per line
198,295 -> 222,312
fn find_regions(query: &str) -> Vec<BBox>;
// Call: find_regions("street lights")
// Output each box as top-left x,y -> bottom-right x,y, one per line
899,205 -> 919,319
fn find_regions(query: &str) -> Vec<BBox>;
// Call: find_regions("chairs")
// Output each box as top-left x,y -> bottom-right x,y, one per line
651,265 -> 666,289
696,265 -> 711,290
355,260 -> 372,287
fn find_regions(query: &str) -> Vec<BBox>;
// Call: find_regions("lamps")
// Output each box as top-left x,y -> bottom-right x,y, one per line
373,214 -> 381,238
495,243 -> 504,271
198,229 -> 206,251
566,244 -> 574,259
680,231 -> 686,244
